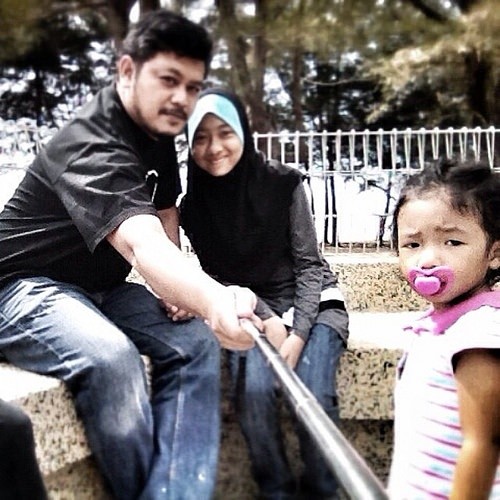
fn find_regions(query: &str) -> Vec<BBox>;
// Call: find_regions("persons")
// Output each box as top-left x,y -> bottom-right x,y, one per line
384,156 -> 500,500
0,10 -> 266,500
177,87 -> 349,500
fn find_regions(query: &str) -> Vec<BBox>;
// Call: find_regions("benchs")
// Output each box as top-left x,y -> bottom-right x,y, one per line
0,311 -> 499,500
322,252 -> 437,312
